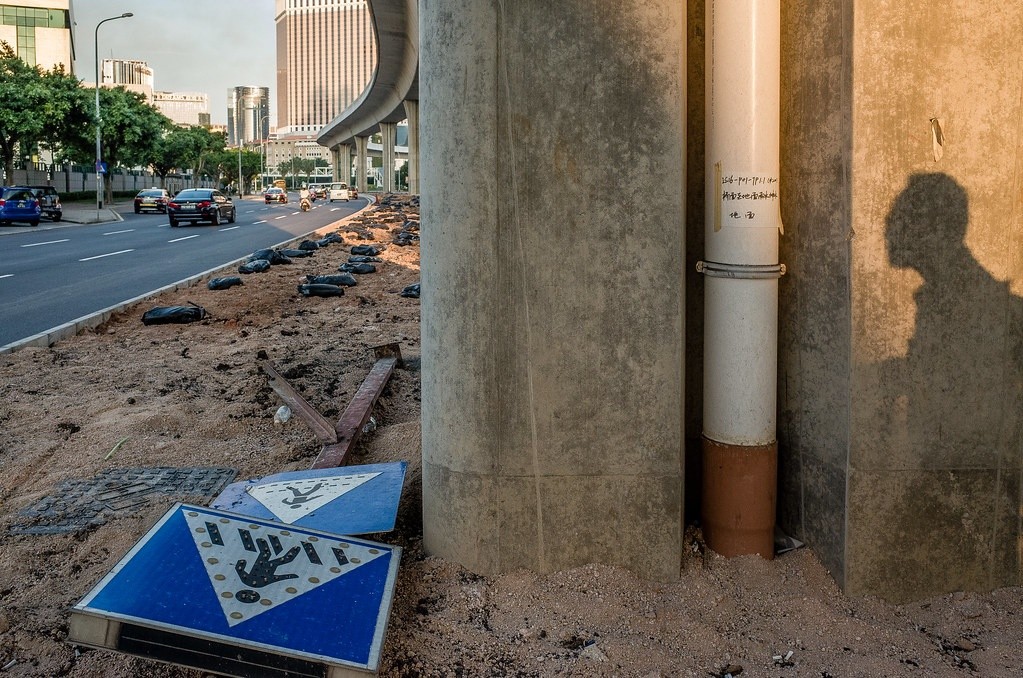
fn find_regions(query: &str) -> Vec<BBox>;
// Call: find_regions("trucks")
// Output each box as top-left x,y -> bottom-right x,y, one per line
272,179 -> 287,193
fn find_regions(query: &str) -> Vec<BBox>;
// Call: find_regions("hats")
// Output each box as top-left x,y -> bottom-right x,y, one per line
302,184 -> 306,188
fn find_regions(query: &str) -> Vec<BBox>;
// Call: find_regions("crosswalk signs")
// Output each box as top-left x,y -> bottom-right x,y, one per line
209,460 -> 409,537
63,499 -> 404,678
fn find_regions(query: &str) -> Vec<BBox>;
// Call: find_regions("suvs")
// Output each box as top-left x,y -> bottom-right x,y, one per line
329,181 -> 350,203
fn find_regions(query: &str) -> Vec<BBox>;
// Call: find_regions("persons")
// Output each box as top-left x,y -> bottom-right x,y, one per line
322,184 -> 327,195
309,186 -> 317,198
299,188 -> 310,202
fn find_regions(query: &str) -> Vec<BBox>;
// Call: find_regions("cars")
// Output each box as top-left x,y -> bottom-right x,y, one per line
0,186 -> 41,227
167,187 -> 237,227
134,186 -> 173,214
347,187 -> 359,199
307,185 -> 327,198
17,184 -> 64,222
265,187 -> 289,204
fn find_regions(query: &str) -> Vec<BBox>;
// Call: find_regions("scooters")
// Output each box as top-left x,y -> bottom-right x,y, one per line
310,192 -> 318,203
298,194 -> 312,212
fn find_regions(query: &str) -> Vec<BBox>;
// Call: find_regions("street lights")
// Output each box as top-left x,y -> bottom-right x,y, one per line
260,115 -> 275,193
94,11 -> 135,209
